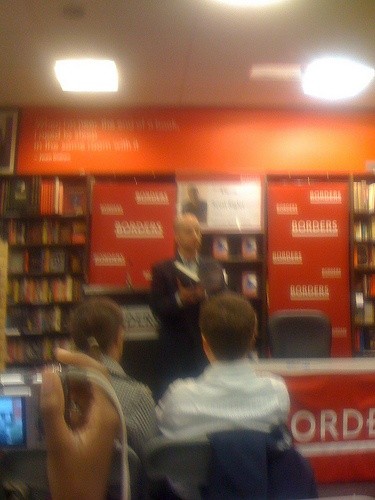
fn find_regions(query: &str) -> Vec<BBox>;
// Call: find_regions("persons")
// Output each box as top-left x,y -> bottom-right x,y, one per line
147,214 -> 225,408
181,184 -> 208,226
0,404 -> 16,442
40,295 -> 291,500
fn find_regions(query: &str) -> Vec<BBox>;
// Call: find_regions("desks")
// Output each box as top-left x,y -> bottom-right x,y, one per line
258,357 -> 375,482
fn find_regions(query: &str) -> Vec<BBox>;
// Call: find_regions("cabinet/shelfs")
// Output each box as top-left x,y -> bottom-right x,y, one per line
1,173 -> 95,372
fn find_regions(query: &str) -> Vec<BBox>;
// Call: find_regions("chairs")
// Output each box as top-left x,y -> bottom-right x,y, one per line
264,309 -> 332,359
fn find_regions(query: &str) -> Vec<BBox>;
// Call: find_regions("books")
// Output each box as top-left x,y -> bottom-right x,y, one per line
1,176 -> 91,212
357,303 -> 374,323
1,220 -> 86,243
355,217 -> 374,242
354,246 -> 374,267
6,338 -> 74,360
8,306 -> 76,331
358,327 -> 374,349
355,274 -> 375,300
354,181 -> 374,212
8,249 -> 84,273
8,277 -> 81,303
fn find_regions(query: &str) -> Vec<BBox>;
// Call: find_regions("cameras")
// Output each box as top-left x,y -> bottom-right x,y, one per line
0,372 -> 72,454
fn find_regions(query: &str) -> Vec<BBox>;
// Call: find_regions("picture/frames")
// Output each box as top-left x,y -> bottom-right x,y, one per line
0,104 -> 22,175
347,171 -> 374,359
